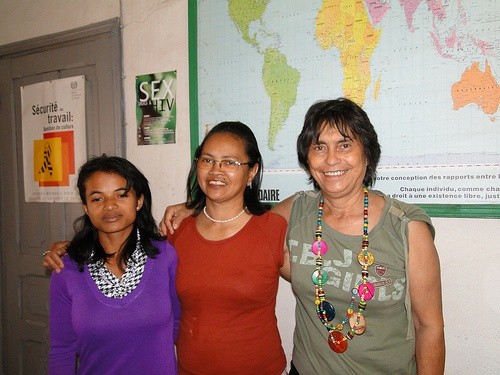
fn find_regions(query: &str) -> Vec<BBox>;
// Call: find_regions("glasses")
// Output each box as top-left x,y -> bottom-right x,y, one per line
195,157 -> 249,171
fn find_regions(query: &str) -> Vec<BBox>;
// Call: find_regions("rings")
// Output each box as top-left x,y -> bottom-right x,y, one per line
42,251 -> 51,256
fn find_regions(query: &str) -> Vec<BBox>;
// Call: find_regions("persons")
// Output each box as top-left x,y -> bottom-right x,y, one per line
46,156 -> 183,374
157,98 -> 447,375
44,120 -> 292,374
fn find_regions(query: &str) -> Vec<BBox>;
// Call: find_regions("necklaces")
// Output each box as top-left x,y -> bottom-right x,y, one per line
203,206 -> 248,223
312,183 -> 376,353
